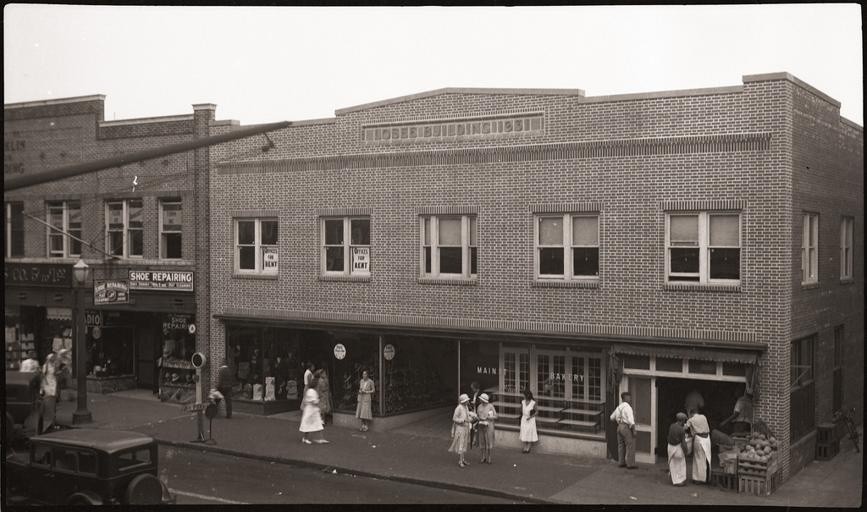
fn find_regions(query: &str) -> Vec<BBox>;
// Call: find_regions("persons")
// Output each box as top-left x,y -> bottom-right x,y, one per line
355,369 -> 376,434
449,393 -> 476,469
683,408 -> 713,485
667,412 -> 687,486
519,389 -> 539,454
40,353 -> 58,418
477,391 -> 497,463
18,349 -> 40,373
468,381 -> 483,447
54,348 -> 75,402
682,382 -> 705,417
299,378 -> 330,445
215,358 -> 232,418
317,368 -> 334,426
717,388 -> 753,436
302,362 -> 317,398
609,392 -> 639,468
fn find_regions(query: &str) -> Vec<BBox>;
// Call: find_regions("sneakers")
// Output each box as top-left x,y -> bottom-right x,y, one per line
360,425 -> 369,433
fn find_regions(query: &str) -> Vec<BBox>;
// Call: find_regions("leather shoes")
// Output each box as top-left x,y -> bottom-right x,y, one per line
302,437 -> 330,445
456,448 -> 531,468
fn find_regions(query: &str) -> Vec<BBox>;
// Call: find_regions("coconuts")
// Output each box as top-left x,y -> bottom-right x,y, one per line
742,432 -> 777,461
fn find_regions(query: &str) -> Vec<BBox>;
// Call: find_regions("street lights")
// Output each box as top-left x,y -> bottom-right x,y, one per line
73,256 -> 88,424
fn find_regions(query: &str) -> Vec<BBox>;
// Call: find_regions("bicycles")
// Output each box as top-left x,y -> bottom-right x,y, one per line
834,405 -> 860,454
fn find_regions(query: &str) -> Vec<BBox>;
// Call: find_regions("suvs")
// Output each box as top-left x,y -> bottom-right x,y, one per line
7,370 -> 170,503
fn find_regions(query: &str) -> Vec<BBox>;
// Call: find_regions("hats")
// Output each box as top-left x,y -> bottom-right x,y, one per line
477,393 -> 489,404
675,412 -> 688,420
457,393 -> 470,404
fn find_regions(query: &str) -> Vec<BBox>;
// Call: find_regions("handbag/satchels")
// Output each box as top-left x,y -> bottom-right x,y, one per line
487,411 -> 493,417
529,409 -> 535,416
